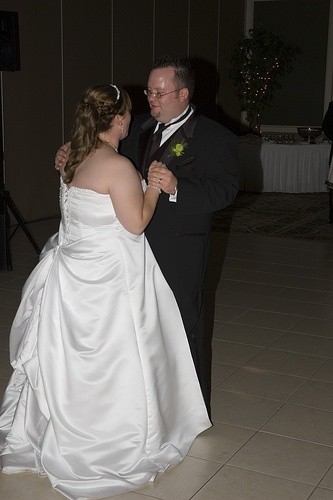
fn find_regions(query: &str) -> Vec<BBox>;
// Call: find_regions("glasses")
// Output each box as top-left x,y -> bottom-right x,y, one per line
143,86 -> 185,99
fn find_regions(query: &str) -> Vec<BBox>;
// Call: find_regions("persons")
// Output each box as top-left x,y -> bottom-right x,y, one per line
53,58 -> 240,426
0,85 -> 213,500
323,101 -> 333,223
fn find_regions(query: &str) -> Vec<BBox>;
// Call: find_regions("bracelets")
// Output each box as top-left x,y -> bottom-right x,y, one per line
147,185 -> 161,194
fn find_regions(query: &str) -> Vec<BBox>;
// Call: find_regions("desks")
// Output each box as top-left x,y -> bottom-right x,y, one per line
239,142 -> 331,193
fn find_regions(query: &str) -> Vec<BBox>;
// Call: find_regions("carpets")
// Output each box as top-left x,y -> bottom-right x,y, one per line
210,192 -> 333,241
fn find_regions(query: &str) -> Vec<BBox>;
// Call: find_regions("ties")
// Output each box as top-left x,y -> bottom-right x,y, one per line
150,104 -> 191,152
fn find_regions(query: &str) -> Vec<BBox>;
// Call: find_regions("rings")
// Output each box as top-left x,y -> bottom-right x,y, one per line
158,179 -> 161,183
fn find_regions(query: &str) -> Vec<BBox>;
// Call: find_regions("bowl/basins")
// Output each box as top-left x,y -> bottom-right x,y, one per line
297,127 -> 323,142
